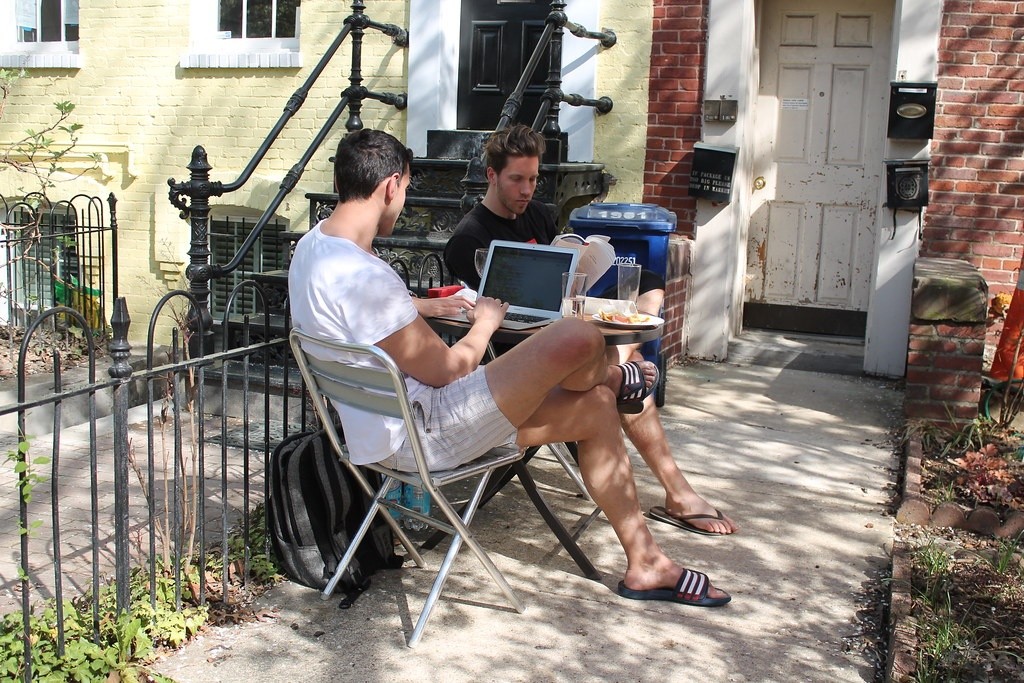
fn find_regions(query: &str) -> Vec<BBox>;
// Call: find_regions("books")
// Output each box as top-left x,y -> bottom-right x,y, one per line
549,233 -> 616,294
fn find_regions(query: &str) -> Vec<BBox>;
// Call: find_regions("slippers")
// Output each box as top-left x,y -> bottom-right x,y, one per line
618,568 -> 731,606
617,401 -> 644,413
649,506 -> 738,536
613,360 -> 660,405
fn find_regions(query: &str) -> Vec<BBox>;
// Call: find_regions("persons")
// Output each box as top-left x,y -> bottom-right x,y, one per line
288,129 -> 731,606
442,125 -> 739,535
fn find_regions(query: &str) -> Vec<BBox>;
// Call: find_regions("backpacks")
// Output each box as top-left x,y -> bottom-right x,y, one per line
264,423 -> 404,608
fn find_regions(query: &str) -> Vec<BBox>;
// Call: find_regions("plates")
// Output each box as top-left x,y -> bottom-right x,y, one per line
592,312 -> 665,330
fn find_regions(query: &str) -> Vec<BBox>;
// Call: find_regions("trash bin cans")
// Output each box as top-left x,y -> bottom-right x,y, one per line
567,201 -> 678,408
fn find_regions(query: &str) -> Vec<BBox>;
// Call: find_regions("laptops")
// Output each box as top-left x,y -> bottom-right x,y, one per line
434,240 -> 581,332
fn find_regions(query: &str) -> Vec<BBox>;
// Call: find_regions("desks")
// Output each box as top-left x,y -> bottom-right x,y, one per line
399,310 -> 666,579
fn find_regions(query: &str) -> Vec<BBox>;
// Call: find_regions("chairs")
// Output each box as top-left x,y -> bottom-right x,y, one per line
291,327 -> 525,649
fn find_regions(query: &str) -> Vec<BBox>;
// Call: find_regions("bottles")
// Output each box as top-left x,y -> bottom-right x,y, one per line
404,482 -> 430,531
381,473 -> 402,524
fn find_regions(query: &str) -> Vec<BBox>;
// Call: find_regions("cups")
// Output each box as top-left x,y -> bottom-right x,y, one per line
474,248 -> 489,278
617,263 -> 642,308
560,272 -> 588,320
